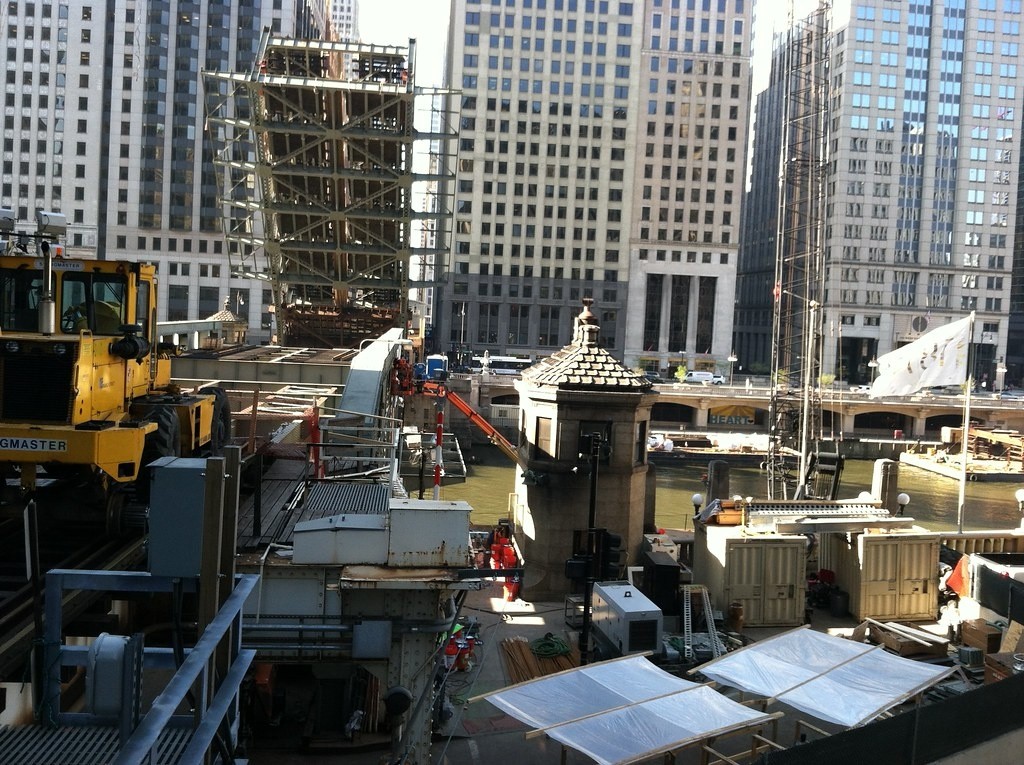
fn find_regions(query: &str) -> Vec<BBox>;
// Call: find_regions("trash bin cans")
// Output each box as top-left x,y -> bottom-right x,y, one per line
894,429 -> 902,440
830,591 -> 850,618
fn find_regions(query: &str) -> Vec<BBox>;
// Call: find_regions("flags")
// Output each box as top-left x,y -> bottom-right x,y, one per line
868,313 -> 972,400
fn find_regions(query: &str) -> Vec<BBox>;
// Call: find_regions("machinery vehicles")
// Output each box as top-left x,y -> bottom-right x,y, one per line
0,206 -> 234,533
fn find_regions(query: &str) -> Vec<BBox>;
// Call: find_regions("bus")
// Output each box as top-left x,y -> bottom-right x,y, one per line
470,356 -> 531,375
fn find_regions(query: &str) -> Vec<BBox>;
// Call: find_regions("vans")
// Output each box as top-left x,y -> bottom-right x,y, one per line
684,372 -> 713,384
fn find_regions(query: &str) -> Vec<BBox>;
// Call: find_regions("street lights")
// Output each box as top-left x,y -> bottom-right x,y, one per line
728,357 -> 737,386
996,367 -> 1008,397
868,360 -> 878,387
679,351 -> 686,364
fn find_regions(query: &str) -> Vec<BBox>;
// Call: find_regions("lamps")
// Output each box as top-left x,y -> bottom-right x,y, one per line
1015,488 -> 1024,511
896,493 -> 910,515
692,494 -> 704,516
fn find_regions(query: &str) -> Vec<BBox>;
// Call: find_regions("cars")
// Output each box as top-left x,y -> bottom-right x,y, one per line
713,375 -> 726,385
646,371 -> 660,377
646,375 -> 665,383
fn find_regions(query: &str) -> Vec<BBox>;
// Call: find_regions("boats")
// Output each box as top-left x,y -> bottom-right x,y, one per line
648,432 -> 802,467
